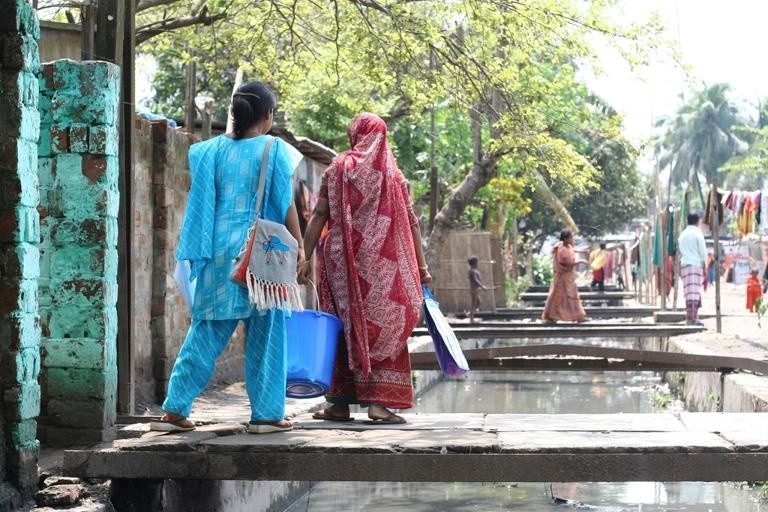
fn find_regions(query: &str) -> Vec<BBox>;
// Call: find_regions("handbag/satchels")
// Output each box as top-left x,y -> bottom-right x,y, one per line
228,218 -> 299,302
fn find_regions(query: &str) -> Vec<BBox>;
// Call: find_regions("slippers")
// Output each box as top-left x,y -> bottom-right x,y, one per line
247,419 -> 293,434
362,412 -> 407,425
312,405 -> 355,422
150,413 -> 196,432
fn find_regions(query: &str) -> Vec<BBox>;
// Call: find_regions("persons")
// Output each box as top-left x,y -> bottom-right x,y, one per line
297,111 -> 432,425
544,213 -> 767,326
149,81 -> 308,436
467,257 -> 487,325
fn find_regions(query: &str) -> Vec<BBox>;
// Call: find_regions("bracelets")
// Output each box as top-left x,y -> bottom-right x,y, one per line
305,259 -> 312,264
419,264 -> 430,273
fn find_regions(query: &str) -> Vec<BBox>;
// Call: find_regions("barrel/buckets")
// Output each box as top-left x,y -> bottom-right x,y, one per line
284,277 -> 343,399
